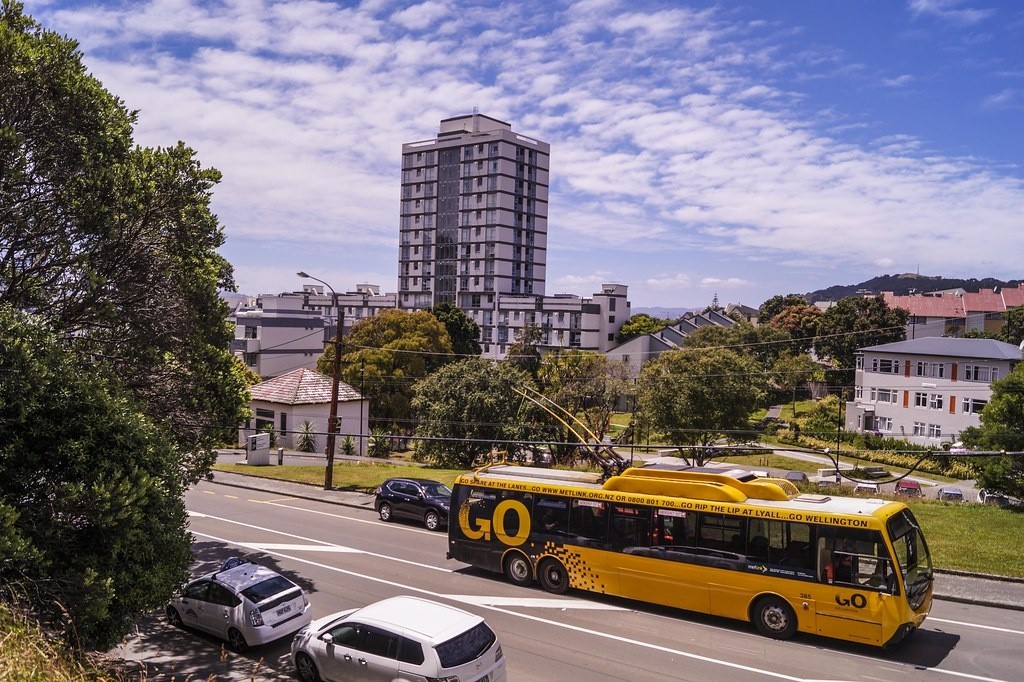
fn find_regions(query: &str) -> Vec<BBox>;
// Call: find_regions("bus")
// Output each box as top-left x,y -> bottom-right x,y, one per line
446,379 -> 935,650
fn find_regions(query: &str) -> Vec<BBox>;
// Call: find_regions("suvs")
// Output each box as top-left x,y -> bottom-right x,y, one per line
785,472 -> 809,490
893,480 -> 927,499
976,488 -> 1009,504
292,594 -> 509,682
374,477 -> 453,531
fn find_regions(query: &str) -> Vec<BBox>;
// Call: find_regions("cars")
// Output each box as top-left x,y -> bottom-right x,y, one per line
935,487 -> 969,504
163,555 -> 311,655
813,481 -> 839,495
853,479 -> 882,496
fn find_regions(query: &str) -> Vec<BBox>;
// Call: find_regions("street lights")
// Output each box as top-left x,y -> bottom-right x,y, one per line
295,270 -> 343,490
